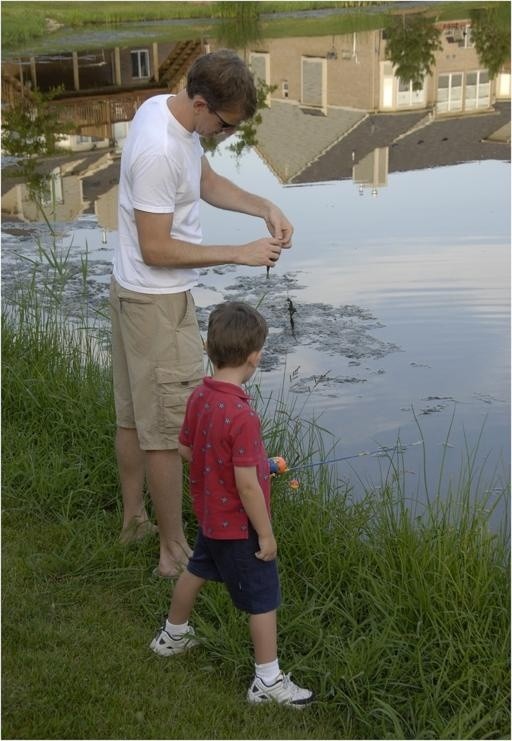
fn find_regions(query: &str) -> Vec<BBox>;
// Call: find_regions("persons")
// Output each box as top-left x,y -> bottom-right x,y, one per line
106,47 -> 296,581
144,301 -> 314,709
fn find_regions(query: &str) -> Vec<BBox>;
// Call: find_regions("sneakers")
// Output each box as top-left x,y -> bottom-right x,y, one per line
149,626 -> 207,657
247,671 -> 314,708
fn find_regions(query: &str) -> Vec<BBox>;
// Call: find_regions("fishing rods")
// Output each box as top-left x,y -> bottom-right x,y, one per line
268,439 -> 424,478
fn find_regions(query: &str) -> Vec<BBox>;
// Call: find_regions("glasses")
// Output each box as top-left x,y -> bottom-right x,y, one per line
211,108 -> 236,130
